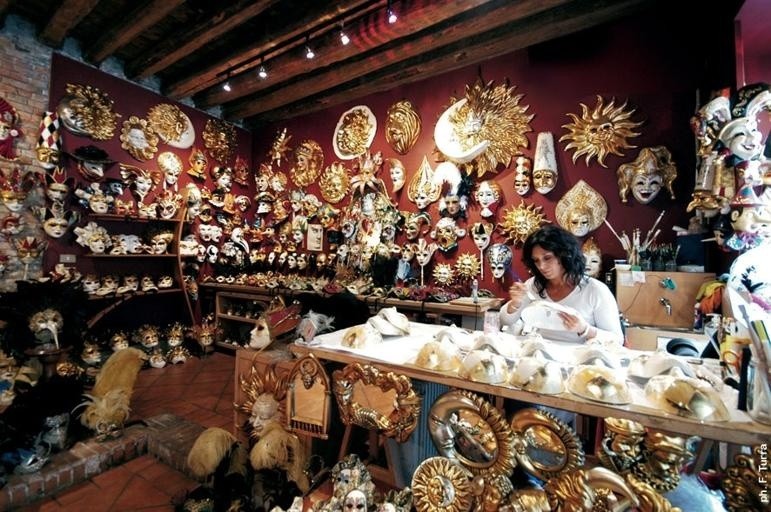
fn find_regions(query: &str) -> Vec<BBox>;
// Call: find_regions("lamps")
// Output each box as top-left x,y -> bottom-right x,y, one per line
216,0 -> 399,94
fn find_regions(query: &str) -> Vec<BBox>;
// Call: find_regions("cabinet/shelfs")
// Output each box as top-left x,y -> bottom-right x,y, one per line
202,276 -> 503,361
80,190 -> 199,357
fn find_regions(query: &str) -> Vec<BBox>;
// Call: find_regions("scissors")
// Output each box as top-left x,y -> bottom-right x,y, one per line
722,350 -> 752,385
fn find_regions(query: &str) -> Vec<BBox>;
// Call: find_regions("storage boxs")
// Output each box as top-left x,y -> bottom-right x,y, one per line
624,325 -> 710,355
616,270 -> 715,327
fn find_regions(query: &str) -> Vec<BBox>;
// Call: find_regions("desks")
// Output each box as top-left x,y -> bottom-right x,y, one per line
289,320 -> 770,490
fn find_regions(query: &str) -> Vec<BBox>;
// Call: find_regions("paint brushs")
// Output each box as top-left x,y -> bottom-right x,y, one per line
738,305 -> 765,363
602,210 -> 666,265
509,270 -> 535,302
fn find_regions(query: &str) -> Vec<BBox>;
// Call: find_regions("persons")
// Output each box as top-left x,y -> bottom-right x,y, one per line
497,226 -> 627,347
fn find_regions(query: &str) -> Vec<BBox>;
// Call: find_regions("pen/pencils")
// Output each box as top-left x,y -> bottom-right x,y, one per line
646,238 -> 682,261
